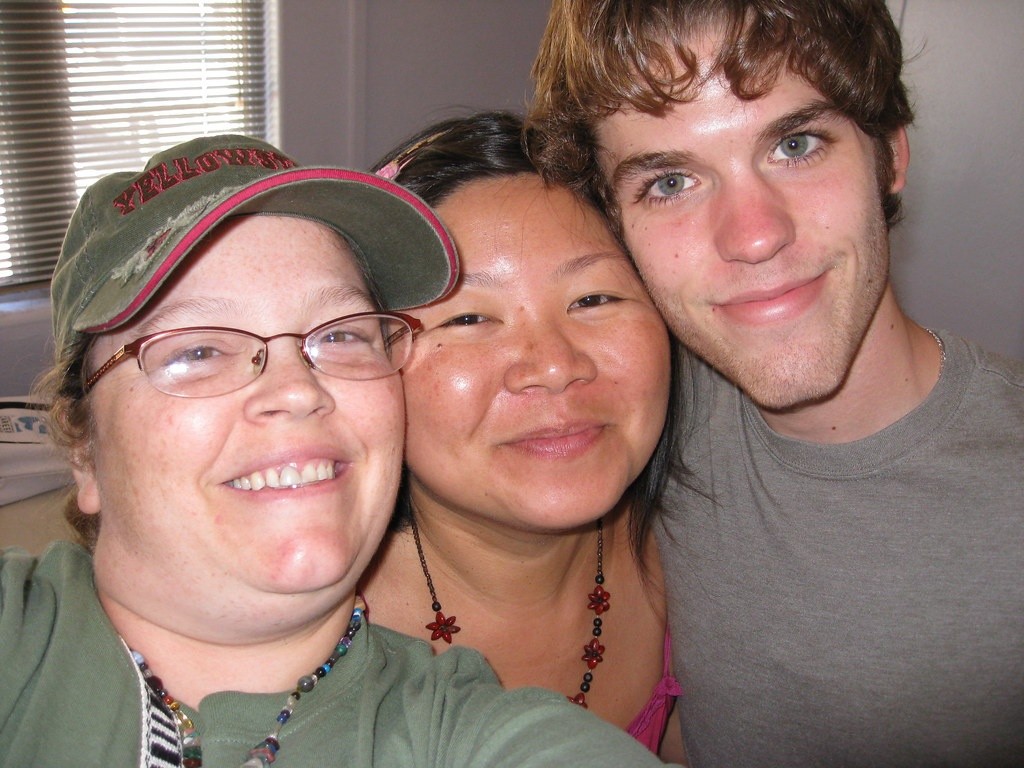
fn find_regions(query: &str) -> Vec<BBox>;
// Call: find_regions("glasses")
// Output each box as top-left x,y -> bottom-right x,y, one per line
76,310 -> 422,399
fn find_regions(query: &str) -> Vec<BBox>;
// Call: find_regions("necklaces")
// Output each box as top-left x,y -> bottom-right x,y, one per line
409,514 -> 610,709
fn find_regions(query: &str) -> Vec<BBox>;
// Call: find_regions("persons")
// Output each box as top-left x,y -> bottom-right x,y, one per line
541,2 -> 1024,768
0,110 -> 690,768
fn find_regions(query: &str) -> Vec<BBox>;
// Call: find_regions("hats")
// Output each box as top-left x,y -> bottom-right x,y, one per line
49,136 -> 459,392
